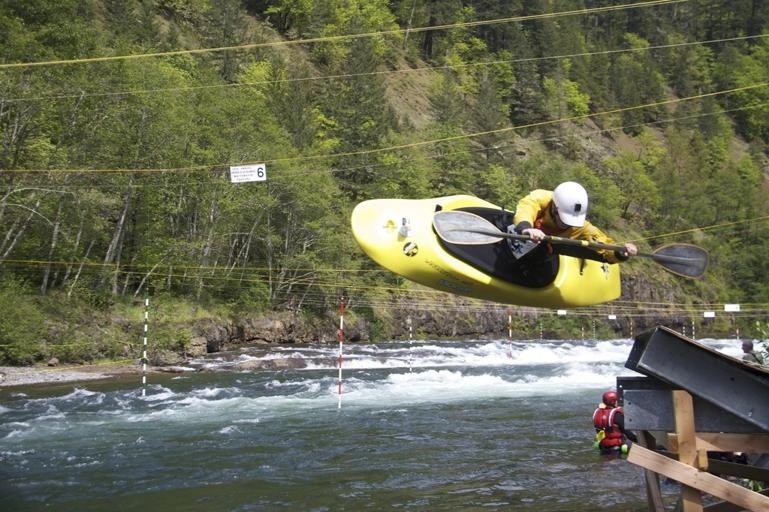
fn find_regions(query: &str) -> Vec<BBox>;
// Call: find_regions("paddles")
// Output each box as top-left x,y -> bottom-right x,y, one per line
432,209 -> 709,280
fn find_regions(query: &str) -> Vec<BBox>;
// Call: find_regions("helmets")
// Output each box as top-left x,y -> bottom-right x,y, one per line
551,180 -> 588,227
602,391 -> 617,405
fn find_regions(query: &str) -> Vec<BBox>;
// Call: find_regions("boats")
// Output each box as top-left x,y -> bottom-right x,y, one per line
350,191 -> 623,309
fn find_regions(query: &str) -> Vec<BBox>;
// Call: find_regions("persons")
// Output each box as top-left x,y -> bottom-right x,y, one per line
497,180 -> 637,282
741,340 -> 764,366
591,391 -> 638,454
